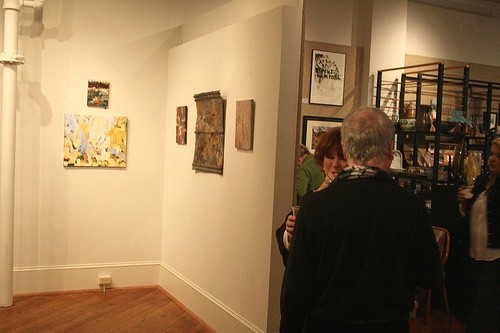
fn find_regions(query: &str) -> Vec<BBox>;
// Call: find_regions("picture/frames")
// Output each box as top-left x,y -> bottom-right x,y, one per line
302,116 -> 345,157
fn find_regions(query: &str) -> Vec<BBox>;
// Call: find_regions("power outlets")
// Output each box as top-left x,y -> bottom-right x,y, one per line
98,276 -> 110,285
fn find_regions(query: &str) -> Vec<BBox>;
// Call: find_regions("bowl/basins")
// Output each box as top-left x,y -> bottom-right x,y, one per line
400,119 -> 416,131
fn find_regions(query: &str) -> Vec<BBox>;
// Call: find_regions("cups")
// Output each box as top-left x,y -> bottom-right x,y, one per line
292,206 -> 300,216
465,187 -> 473,199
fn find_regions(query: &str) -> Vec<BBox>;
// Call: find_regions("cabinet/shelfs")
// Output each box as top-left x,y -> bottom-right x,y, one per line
376,63 -> 500,228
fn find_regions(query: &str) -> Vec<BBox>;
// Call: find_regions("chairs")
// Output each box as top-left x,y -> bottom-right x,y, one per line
419,227 -> 451,325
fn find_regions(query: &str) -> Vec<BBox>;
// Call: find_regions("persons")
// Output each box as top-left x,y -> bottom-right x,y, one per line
456,138 -> 500,333
280,107 -> 441,333
296,144 -> 325,203
275,128 -> 349,270
